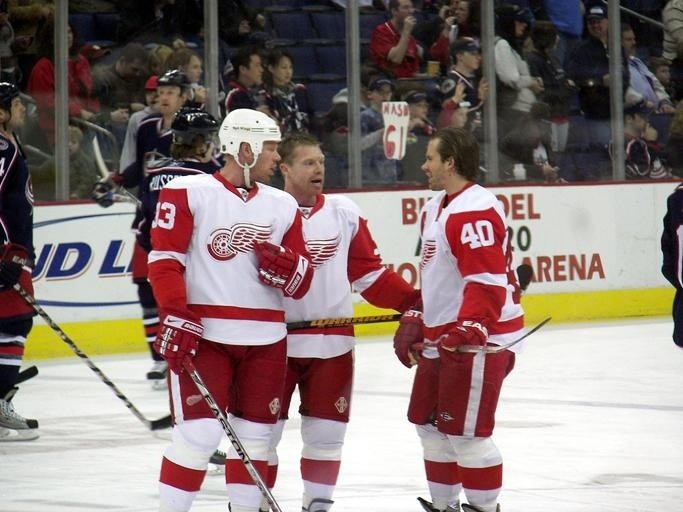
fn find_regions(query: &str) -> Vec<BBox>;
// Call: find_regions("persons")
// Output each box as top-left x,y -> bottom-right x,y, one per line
145,108 -> 314,511
0,81 -> 39,431
129,107 -> 220,255
1,0 -> 683,201
661,183 -> 683,348
91,68 -> 191,379
259,134 -> 422,512
393,125 -> 524,512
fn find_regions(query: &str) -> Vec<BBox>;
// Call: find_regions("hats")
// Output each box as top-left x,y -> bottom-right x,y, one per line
368,79 -> 395,91
498,4 -> 530,18
143,75 -> 158,89
584,5 -> 608,20
79,44 -> 112,62
623,97 -> 654,114
450,37 -> 481,53
402,92 -> 433,105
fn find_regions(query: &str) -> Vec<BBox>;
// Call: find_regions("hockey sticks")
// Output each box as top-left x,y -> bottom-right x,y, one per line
285,265 -> 533,332
9,283 -> 173,430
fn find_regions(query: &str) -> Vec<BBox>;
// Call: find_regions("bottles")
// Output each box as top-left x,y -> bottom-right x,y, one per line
513,164 -> 525,180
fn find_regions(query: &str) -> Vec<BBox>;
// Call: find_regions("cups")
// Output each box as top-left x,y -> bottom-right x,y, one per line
428,61 -> 440,75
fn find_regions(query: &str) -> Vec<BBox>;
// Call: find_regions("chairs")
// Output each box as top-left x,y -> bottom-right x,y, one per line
65,5 -> 676,190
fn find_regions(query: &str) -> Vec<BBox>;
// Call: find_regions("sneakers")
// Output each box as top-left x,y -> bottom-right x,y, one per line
146,360 -> 168,380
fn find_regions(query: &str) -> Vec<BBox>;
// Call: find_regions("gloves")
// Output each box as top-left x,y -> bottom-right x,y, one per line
437,321 -> 491,367
151,305 -> 203,377
251,238 -> 313,299
90,170 -> 127,208
392,309 -> 427,369
0,243 -> 31,293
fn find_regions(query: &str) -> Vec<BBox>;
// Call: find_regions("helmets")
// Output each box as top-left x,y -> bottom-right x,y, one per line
216,108 -> 282,158
0,82 -> 19,104
169,108 -> 221,134
156,69 -> 191,90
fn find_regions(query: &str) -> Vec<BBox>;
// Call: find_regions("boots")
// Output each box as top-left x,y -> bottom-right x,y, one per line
0,386 -> 37,429
